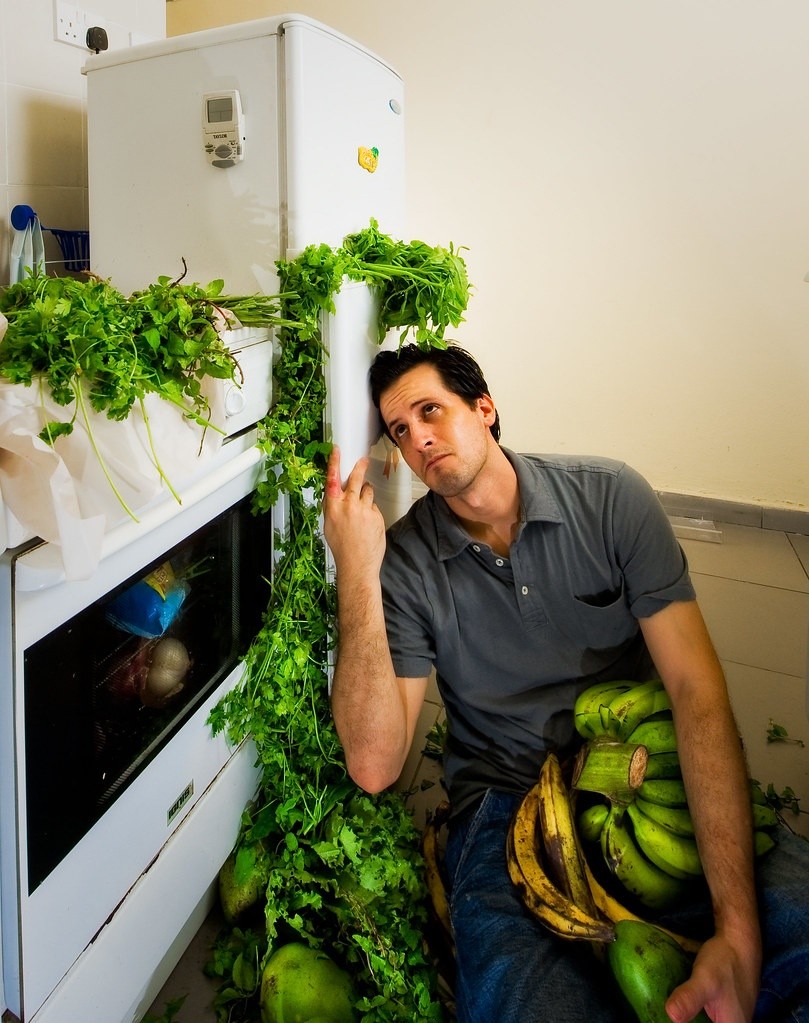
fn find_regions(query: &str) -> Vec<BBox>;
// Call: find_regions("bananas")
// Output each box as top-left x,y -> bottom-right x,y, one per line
505,677 -> 781,966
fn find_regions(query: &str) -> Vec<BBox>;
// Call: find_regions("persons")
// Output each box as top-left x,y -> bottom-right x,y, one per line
322,344 -> 809,1023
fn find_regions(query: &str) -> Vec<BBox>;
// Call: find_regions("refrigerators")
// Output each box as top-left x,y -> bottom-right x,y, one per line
81,15 -> 413,697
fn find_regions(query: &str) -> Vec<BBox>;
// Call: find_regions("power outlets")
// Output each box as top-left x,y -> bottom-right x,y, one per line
53,0 -> 131,55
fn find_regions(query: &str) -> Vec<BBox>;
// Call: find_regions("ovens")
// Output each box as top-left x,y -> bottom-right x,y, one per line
0,416 -> 285,1023
